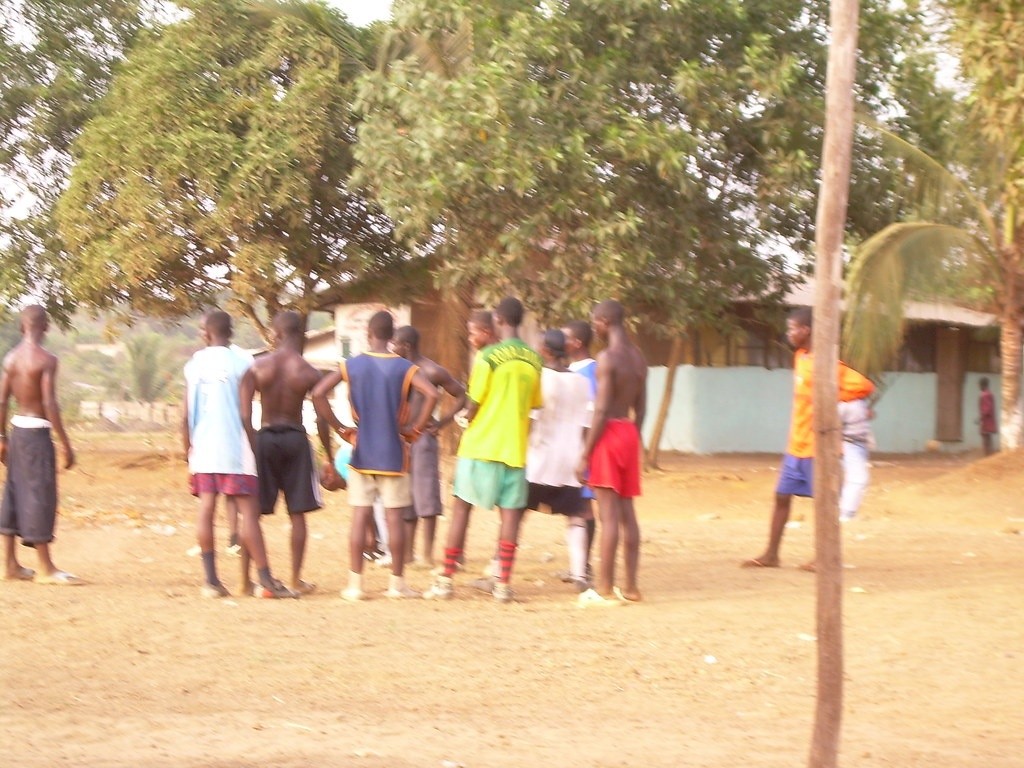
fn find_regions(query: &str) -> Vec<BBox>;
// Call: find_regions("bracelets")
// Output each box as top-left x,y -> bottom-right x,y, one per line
0,434 -> 7,440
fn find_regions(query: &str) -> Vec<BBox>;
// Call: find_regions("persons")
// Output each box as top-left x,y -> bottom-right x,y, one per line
184,311 -> 300,600
389,323 -> 466,569
312,309 -> 437,600
489,327 -> 591,594
745,310 -> 874,572
975,376 -> 996,457
418,297 -> 544,600
0,305 -> 86,587
560,320 -> 602,581
575,301 -> 649,606
237,310 -> 348,596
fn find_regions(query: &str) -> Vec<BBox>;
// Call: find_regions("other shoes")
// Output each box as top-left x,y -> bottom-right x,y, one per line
574,562 -> 592,591
423,574 -> 456,600
340,571 -> 366,600
492,580 -> 512,600
360,546 -> 393,565
256,578 -> 299,598
381,576 -> 406,597
204,583 -> 231,599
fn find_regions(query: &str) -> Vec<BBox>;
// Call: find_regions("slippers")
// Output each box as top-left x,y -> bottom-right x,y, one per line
739,558 -> 780,569
612,586 -> 639,604
40,571 -> 82,586
577,588 -> 624,607
1,566 -> 37,582
